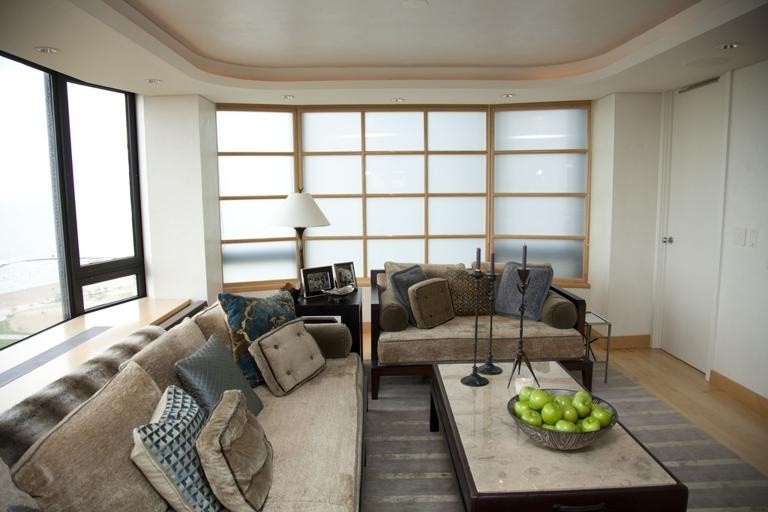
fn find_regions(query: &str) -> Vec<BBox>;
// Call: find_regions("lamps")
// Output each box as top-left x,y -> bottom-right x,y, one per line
271,186 -> 331,289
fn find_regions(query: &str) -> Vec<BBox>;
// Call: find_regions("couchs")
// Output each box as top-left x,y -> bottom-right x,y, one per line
0,301 -> 367,512
371,262 -> 593,398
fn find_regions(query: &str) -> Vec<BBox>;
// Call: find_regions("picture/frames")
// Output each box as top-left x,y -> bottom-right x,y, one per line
301,266 -> 335,297
333,262 -> 357,290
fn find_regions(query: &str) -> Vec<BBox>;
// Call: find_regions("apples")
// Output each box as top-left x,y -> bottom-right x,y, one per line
513,384 -> 612,433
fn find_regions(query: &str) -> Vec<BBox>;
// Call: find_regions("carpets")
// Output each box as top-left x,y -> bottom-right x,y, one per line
361,356 -> 768,511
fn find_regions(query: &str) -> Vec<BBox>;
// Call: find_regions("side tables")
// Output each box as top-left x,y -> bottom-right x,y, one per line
584,310 -> 612,384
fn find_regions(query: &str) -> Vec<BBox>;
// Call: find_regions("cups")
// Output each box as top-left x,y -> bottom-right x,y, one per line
514,373 -> 534,395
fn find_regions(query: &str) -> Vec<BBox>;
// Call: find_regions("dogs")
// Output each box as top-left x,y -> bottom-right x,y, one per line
336,268 -> 352,288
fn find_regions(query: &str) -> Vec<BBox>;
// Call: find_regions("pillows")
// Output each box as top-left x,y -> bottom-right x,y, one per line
217,290 -> 299,388
195,387 -> 276,511
116,316 -> 209,390
379,262 -> 578,332
247,317 -> 328,398
191,301 -> 236,356
130,383 -> 221,512
173,331 -> 268,420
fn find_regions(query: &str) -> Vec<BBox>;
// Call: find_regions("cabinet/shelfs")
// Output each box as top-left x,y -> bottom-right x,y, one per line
295,287 -> 363,360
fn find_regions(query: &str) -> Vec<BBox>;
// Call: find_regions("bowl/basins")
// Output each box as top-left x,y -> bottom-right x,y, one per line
508,388 -> 617,450
